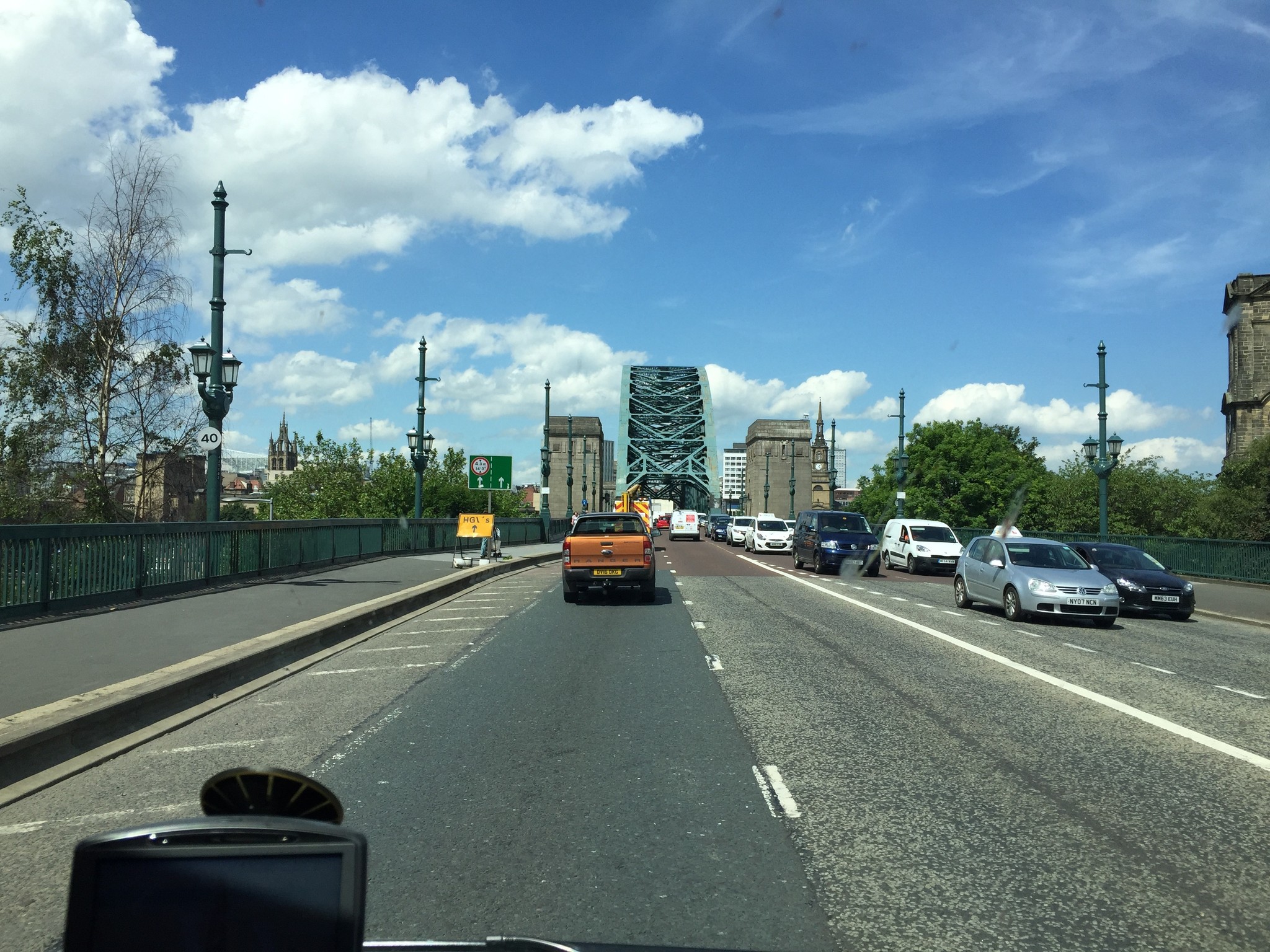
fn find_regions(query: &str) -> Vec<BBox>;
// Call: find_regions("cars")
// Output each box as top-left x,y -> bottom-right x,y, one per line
744,513 -> 793,554
784,520 -> 796,538
725,515 -> 756,547
1063,541 -> 1196,621
699,515 -> 706,527
657,515 -> 672,530
952,523 -> 1120,626
710,517 -> 730,541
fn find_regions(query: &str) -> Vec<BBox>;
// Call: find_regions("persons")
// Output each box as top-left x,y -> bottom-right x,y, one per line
481,509 -> 497,557
585,510 -> 589,513
904,531 -> 921,540
622,519 -> 632,532
1112,551 -> 1123,563
570,512 -> 580,527
822,516 -> 829,526
1085,551 -> 1097,562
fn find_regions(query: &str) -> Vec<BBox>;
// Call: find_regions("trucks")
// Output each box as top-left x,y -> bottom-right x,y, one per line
668,509 -> 700,541
697,513 -> 706,525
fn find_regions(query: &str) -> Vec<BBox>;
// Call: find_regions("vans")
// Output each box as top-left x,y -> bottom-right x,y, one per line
880,518 -> 966,578
792,510 -> 880,577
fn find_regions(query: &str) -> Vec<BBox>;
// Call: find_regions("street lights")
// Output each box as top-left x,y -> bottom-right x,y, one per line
406,335 -> 442,517
564,412 -> 598,513
886,387 -> 914,517
536,377 -> 554,521
821,417 -> 841,509
720,467 -> 748,516
760,450 -> 771,513
788,438 -> 798,520
1080,341 -> 1125,535
187,180 -> 254,522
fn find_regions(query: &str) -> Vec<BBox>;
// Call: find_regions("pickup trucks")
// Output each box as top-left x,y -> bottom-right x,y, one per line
560,512 -> 662,598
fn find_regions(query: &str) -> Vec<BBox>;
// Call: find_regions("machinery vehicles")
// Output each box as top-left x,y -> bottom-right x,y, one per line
612,484 -> 654,538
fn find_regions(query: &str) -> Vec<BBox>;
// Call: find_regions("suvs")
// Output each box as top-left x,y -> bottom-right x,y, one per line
704,513 -> 730,538
